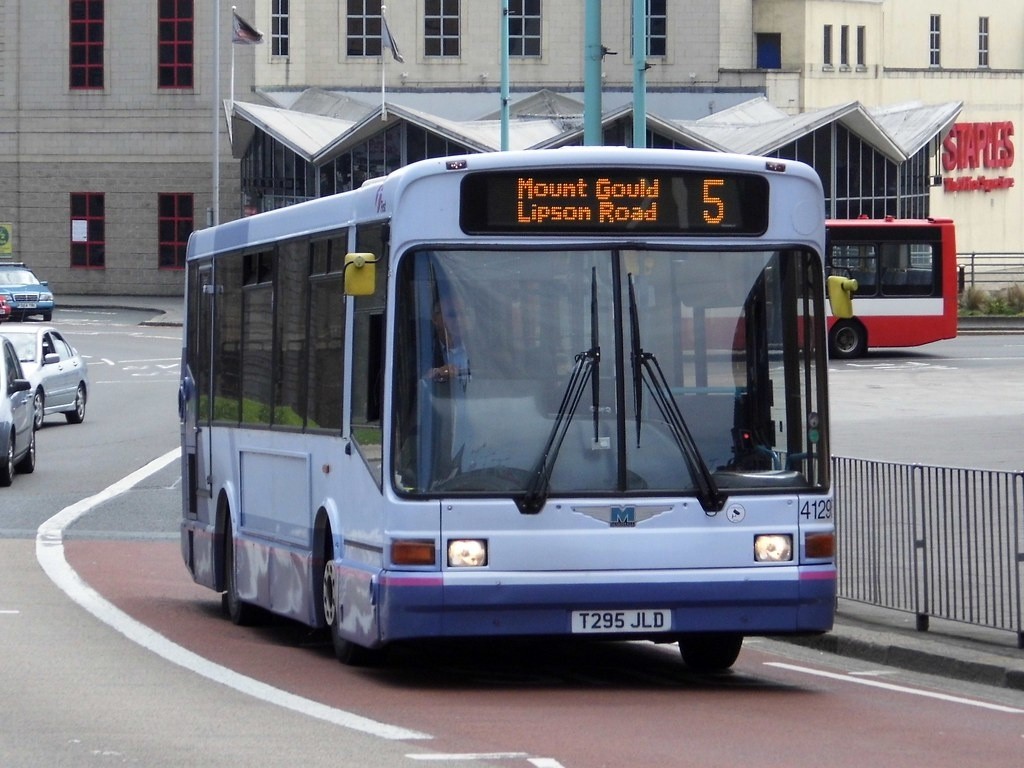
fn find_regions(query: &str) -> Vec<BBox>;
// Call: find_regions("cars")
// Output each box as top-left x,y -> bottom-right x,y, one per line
0,336 -> 38,487
0,295 -> 12,324
1,322 -> 88,430
0,261 -> 55,321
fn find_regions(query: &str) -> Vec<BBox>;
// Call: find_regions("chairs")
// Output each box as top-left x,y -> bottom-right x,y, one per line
221,335 -> 348,431
847,266 -> 934,287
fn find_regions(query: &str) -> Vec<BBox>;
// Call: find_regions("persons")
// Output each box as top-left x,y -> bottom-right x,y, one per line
417,287 -> 523,382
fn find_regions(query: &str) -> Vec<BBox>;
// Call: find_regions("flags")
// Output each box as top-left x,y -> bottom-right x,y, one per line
382,16 -> 405,64
232,12 -> 266,45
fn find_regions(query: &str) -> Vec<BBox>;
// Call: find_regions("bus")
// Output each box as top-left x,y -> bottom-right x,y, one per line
503,216 -> 965,361
175,143 -> 862,688
